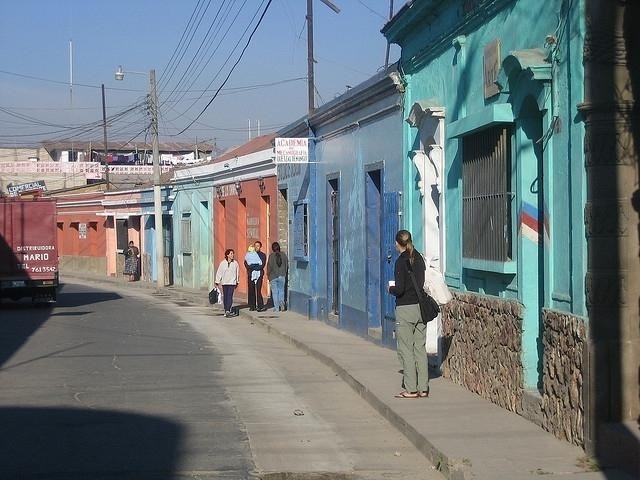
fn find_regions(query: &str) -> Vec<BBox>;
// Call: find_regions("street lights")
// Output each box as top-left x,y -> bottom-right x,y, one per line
114,63 -> 165,290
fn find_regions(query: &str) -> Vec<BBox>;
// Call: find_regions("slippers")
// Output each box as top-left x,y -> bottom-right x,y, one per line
417,390 -> 428,398
394,391 -> 419,398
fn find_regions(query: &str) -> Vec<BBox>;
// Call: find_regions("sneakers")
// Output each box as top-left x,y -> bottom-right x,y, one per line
225,311 -> 231,316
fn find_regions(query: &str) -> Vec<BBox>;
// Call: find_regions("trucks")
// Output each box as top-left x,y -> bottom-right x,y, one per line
0,194 -> 59,308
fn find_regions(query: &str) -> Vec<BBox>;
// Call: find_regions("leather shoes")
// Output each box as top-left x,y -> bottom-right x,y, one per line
249,307 -> 254,310
256,307 -> 261,311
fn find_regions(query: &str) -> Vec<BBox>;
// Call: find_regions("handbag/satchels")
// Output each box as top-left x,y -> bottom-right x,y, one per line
417,292 -> 440,324
423,265 -> 453,308
208,290 -> 219,305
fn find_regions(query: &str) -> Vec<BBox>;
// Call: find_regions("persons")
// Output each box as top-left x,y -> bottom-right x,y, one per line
214,249 -> 240,317
243,241 -> 266,312
387,230 -> 429,399
245,243 -> 263,285
267,242 -> 288,312
122,240 -> 140,282
